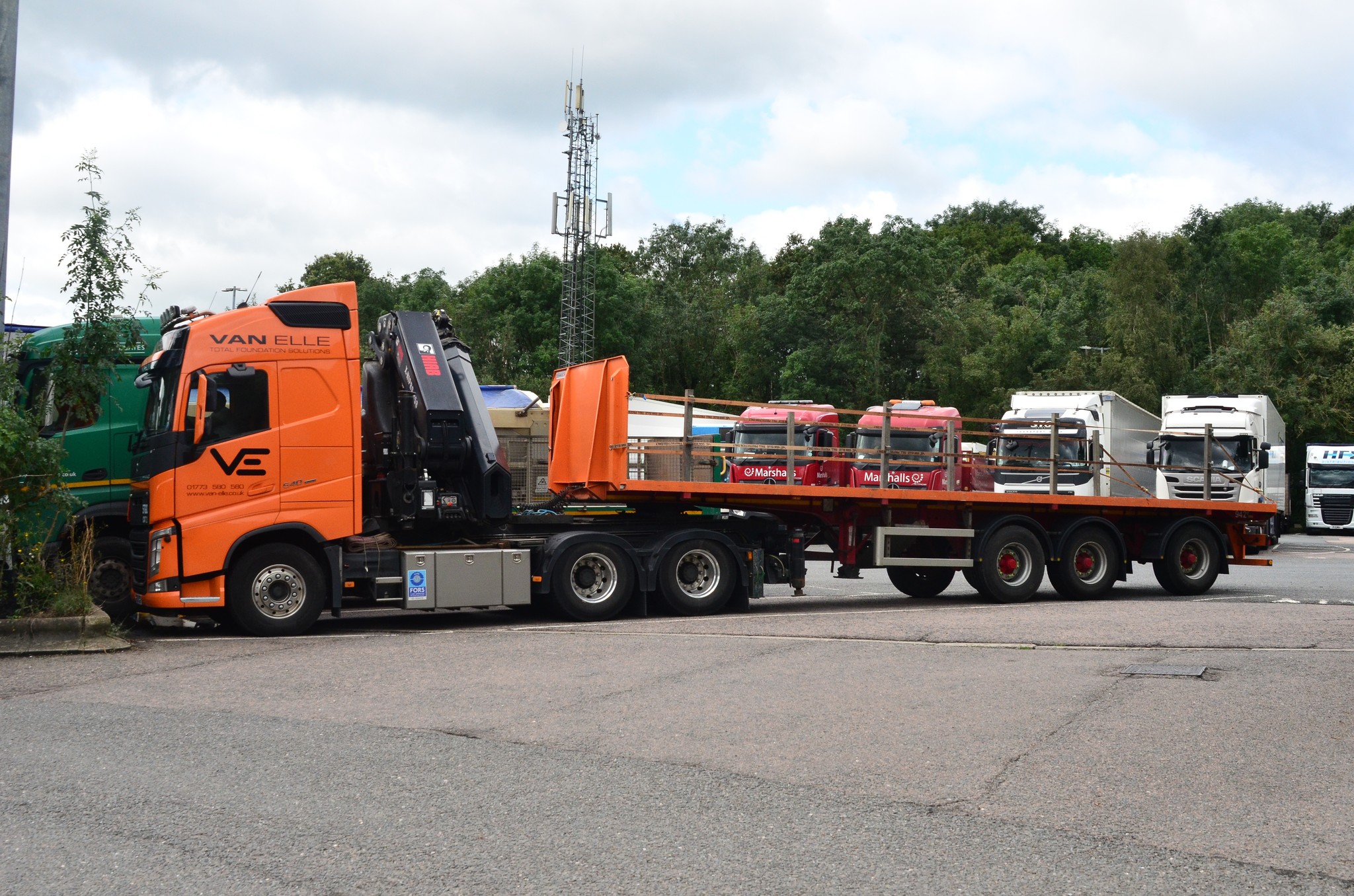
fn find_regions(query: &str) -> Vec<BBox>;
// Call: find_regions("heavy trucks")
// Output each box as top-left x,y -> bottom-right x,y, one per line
723,391 -> 848,487
127,286 -> 1279,638
846,399 -> 996,492
0,319 -> 153,622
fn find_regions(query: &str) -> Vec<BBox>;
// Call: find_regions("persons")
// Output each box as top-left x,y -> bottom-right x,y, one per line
203,391 -> 241,442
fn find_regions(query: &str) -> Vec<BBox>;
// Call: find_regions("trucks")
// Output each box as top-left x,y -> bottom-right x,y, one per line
1304,443 -> 1354,535
1145,392 -> 1287,539
984,384 -> 1159,499
